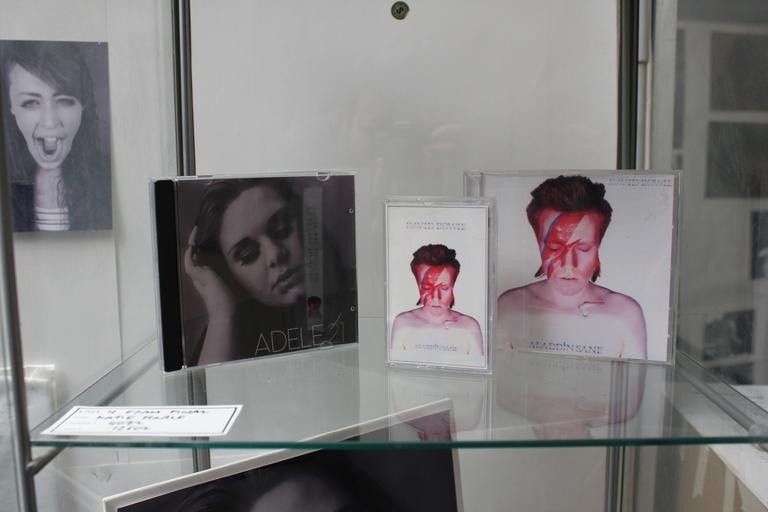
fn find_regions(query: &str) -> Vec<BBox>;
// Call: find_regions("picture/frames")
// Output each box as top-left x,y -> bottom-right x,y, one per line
461,169 -> 681,370
102,398 -> 463,512
383,193 -> 500,372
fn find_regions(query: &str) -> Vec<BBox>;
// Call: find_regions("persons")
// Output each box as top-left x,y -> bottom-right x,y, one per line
497,175 -> 647,359
1,40 -> 112,231
183,181 -> 358,369
391,373 -> 483,440
178,459 -> 403,511
307,296 -> 321,319
497,350 -> 646,440
390,242 -> 484,367
